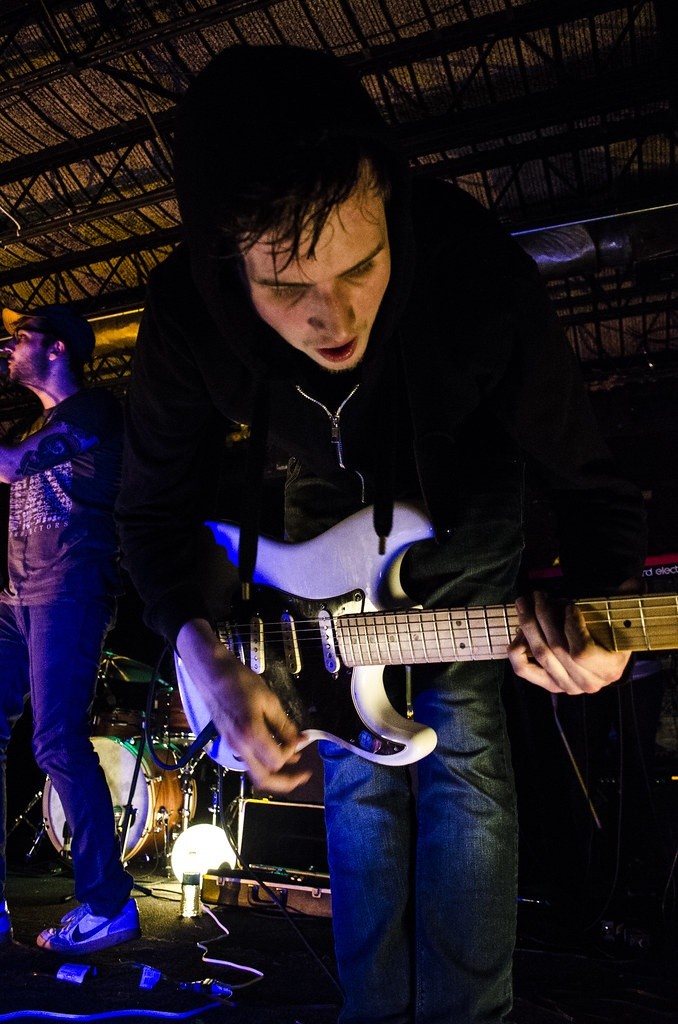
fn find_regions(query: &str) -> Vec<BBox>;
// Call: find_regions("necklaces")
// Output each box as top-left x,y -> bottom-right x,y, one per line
27,410 -> 53,436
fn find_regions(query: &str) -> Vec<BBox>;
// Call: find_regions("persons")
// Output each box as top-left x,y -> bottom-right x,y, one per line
0,307 -> 141,958
116,42 -> 649,1024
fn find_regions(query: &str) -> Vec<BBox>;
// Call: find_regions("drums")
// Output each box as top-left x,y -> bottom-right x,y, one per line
43,735 -> 197,864
90,706 -> 141,740
157,687 -> 191,730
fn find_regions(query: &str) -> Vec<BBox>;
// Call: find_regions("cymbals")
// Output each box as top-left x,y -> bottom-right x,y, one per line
99,651 -> 155,681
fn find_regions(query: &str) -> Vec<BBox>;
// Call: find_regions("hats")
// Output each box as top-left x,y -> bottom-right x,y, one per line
2,304 -> 96,357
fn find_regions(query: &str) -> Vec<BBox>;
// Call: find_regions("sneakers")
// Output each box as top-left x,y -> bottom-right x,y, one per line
0,899 -> 14,942
36,898 -> 140,956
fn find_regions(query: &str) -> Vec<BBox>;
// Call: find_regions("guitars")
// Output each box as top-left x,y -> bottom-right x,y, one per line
177,493 -> 678,772
